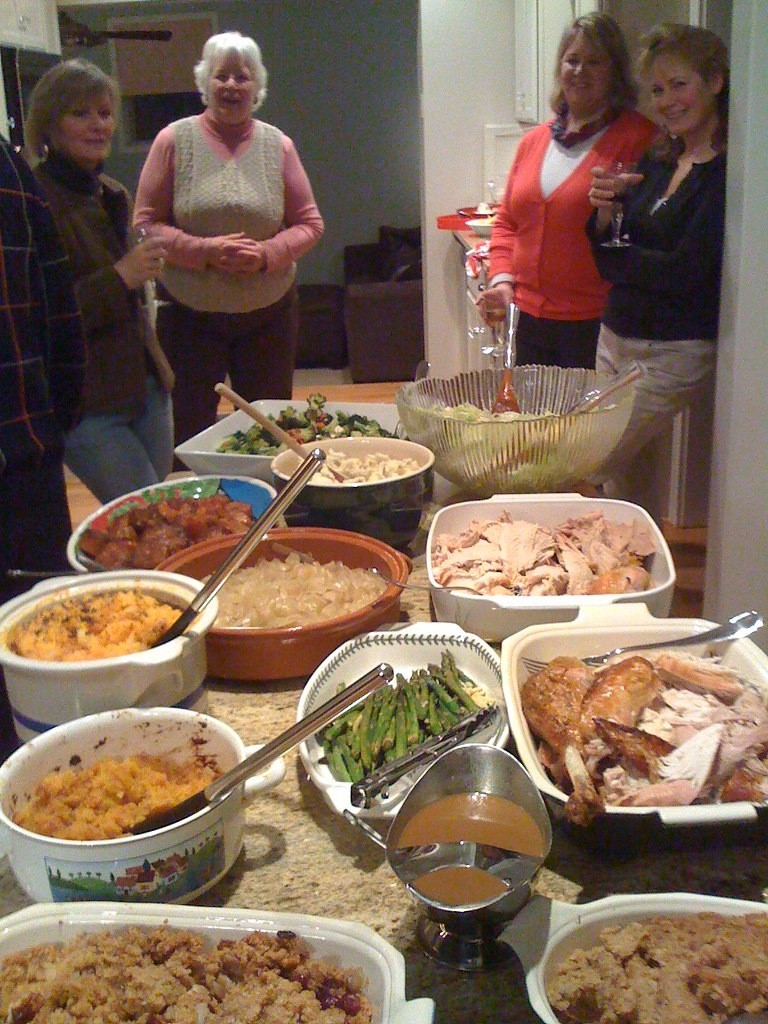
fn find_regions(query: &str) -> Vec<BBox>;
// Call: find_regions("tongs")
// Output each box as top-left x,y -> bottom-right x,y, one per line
350,702 -> 500,809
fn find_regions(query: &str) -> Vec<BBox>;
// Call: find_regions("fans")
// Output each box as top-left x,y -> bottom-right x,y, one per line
58,10 -> 172,48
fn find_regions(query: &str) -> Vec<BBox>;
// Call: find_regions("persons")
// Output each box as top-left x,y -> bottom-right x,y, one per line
0,56 -> 175,765
585,22 -> 730,531
133,32 -> 324,473
475,11 -> 664,369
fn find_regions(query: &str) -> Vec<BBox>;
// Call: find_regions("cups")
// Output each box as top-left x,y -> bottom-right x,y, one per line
342,743 -> 553,972
477,203 -> 492,214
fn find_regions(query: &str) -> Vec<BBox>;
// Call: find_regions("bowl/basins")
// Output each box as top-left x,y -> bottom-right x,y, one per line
465,219 -> 495,238
270,438 -> 435,548
67,475 -> 279,572
427,494 -> 677,642
503,606 -> 767,1023
173,400 -> 409,489
155,527 -> 412,681
0,899 -> 435,1024
397,364 -> 636,497
0,569 -> 286,904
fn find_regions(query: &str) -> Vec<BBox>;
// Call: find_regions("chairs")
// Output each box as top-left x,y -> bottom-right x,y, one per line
343,242 -> 425,384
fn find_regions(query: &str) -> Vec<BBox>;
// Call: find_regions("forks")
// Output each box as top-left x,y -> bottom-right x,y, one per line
521,610 -> 764,676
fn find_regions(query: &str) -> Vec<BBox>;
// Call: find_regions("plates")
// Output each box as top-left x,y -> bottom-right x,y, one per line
298,622 -> 509,819
456,206 -> 500,219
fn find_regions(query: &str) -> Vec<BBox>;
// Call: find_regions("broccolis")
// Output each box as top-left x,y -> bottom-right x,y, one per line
218,392 -> 398,456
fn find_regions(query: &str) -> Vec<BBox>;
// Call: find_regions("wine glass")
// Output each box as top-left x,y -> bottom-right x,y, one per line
481,295 -> 508,358
601,161 -> 637,248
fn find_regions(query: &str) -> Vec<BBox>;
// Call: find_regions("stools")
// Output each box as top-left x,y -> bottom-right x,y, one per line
295,284 -> 347,369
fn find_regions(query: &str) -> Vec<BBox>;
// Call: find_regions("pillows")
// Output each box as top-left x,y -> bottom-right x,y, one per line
372,225 -> 421,281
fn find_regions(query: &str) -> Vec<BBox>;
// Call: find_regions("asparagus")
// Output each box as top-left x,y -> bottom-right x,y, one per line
318,650 -> 485,782
